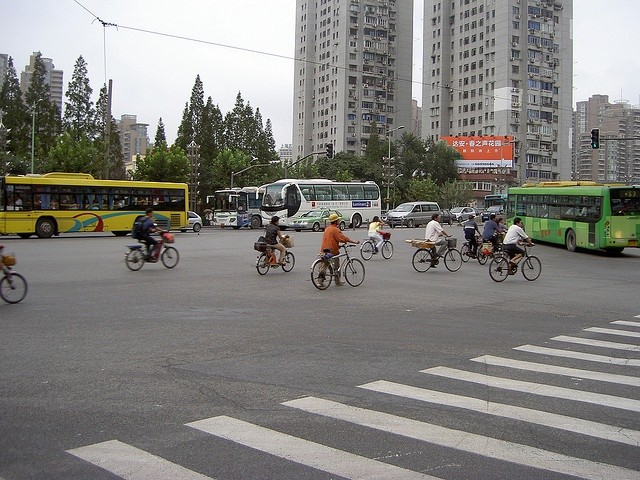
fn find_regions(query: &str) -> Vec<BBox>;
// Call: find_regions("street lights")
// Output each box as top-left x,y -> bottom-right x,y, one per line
25,98 -> 43,174
381,125 -> 404,209
249,157 -> 258,164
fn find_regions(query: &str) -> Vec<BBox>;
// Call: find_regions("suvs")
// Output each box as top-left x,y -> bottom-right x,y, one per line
480,205 -> 503,222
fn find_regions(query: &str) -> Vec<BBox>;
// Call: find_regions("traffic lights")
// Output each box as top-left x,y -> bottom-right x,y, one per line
590,128 -> 600,149
326,143 -> 334,160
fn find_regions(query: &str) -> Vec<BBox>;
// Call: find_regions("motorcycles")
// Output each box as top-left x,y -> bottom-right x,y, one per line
0,245 -> 28,304
121,220 -> 180,270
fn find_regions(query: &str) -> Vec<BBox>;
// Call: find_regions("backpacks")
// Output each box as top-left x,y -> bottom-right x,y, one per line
131,217 -> 150,238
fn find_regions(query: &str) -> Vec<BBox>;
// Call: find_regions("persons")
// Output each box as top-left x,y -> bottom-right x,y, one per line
482,214 -> 502,254
503,217 -> 534,275
8,192 -> 23,209
368,216 -> 385,253
153,197 -> 158,205
425,214 -> 453,268
316,214 -> 360,287
463,215 -> 482,257
497,218 -> 508,235
204,211 -> 209,225
116,200 -> 123,205
93,198 -> 100,203
131,208 -> 168,261
237,204 -> 243,210
208,211 -> 213,225
265,216 -> 287,265
136,195 -> 145,205
69,198 -> 78,209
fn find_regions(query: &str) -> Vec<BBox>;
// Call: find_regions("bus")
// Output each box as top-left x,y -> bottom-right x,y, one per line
481,194 -> 507,213
206,186 -> 266,229
503,181 -> 640,253
0,172 -> 189,238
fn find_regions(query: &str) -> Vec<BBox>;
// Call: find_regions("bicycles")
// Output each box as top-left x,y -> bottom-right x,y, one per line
360,232 -> 394,261
307,241 -> 365,290
255,234 -> 295,275
460,233 -> 482,262
475,234 -> 504,265
489,243 -> 541,282
412,235 -> 462,273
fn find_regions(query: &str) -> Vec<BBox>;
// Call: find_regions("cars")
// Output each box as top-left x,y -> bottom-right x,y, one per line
440,209 -> 457,225
381,209 -> 393,225
289,206 -> 351,232
180,210 -> 203,232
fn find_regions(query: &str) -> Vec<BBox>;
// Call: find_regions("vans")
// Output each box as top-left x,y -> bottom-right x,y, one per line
450,206 -> 476,223
387,201 -> 441,228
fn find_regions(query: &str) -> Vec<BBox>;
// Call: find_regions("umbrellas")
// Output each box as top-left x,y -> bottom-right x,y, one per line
203,209 -> 213,213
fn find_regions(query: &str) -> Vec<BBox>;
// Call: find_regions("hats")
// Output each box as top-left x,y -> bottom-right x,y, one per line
328,214 -> 342,222
468,215 -> 475,219
